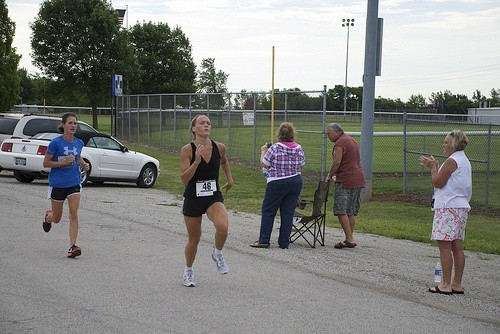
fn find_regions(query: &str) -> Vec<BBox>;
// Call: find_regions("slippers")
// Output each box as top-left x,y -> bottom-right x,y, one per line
452,290 -> 464,294
334,240 -> 357,249
429,286 -> 453,295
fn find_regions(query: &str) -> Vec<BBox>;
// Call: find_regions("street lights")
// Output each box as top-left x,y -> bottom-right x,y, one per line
342,19 -> 355,122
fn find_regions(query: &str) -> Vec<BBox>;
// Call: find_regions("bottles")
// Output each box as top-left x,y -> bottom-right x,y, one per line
434,262 -> 441,282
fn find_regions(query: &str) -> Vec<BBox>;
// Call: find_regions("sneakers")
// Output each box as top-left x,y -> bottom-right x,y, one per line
212,251 -> 229,275
67,245 -> 81,257
182,269 -> 196,287
42,210 -> 52,233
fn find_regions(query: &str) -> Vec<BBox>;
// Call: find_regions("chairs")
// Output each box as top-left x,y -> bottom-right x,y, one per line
289,181 -> 329,248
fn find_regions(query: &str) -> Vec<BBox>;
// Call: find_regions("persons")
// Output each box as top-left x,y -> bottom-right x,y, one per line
325,123 -> 366,249
42,112 -> 89,258
180,114 -> 234,287
250,122 -> 305,249
418,130 -> 473,296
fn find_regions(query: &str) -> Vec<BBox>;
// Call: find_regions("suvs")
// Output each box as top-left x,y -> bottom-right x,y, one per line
0,112 -> 119,149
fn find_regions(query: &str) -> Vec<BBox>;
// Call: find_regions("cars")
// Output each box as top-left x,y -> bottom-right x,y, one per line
0,130 -> 160,188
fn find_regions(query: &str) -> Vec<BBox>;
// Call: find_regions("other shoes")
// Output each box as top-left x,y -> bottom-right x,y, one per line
250,241 -> 270,248
